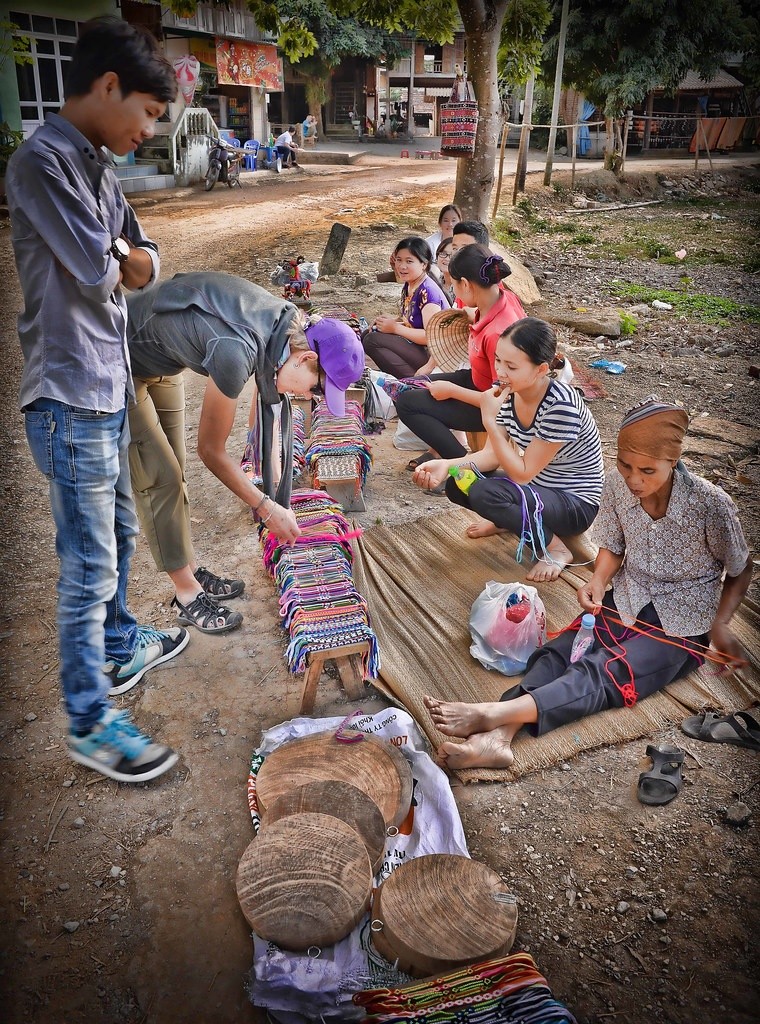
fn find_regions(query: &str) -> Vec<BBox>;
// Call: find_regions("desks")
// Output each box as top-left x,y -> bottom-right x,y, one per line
233,147 -> 256,157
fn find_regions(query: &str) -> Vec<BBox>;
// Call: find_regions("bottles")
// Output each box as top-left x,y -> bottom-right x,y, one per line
570,613 -> 595,663
376,377 -> 407,402
359,316 -> 368,333
449,465 -> 479,496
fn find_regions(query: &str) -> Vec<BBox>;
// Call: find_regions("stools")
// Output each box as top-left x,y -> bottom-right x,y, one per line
415,150 -> 431,160
431,150 -> 447,160
400,150 -> 409,158
263,147 -> 279,162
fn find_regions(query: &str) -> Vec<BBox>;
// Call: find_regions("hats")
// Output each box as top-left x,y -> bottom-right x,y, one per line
426,309 -> 475,374
305,318 -> 365,418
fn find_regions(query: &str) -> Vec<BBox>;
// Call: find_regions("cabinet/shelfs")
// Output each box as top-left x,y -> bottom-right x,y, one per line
204,95 -> 227,128
229,113 -> 249,140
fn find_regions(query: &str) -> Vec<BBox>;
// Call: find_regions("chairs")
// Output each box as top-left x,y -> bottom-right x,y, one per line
299,125 -> 315,149
244,138 -> 260,169
227,139 -> 240,151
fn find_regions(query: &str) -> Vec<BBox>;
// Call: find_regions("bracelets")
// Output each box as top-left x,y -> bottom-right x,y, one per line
262,502 -> 276,523
253,494 -> 269,512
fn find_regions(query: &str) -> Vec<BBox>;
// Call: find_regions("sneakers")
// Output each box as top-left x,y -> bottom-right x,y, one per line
69,708 -> 179,782
100,624 -> 190,696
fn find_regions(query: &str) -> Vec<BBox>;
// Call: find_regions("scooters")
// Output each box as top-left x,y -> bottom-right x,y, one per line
204,135 -> 242,191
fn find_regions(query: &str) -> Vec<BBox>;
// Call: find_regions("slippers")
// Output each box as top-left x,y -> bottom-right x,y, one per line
680,711 -> 760,747
406,452 -> 435,471
638,744 -> 684,805
423,478 -> 447,497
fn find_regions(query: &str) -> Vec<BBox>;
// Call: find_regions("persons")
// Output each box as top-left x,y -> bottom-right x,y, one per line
361,238 -> 451,380
303,114 -> 317,138
436,220 -> 490,309
276,126 -> 299,168
424,393 -> 752,770
5,16 -> 190,782
412,317 -> 605,581
124,272 -> 366,633
394,243 -> 529,496
424,204 -> 462,261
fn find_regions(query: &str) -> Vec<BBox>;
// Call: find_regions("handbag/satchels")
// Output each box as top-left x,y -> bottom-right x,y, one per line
440,77 -> 479,157
469,580 -> 546,676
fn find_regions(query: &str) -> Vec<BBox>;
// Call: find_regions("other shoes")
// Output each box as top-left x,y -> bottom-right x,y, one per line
292,162 -> 299,168
283,165 -> 290,168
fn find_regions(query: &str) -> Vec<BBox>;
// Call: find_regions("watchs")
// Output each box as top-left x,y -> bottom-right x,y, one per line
110,237 -> 130,263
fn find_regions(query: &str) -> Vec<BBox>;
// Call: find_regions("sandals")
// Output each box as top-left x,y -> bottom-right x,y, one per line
195,567 -> 243,598
171,591 -> 243,633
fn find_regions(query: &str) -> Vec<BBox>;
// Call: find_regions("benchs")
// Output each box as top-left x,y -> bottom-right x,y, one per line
259,488 -> 379,716
247,405 -> 304,526
312,396 -> 368,514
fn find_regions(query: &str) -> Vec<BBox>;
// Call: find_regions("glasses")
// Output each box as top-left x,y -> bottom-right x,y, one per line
438,252 -> 452,258
310,339 -> 324,396
